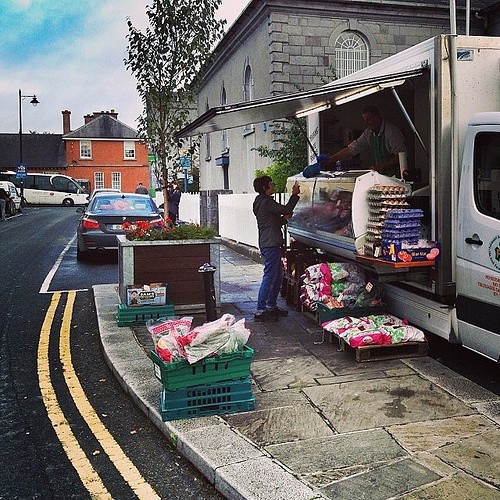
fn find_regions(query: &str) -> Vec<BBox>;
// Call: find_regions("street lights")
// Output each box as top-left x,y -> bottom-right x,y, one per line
18,88 -> 41,165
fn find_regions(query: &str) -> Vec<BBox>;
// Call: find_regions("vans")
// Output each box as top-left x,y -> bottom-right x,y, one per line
1,181 -> 22,213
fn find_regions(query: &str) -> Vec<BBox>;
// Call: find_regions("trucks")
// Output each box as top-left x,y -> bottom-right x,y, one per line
178,31 -> 500,367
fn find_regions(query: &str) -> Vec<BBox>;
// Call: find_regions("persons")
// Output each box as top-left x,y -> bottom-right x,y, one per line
167,182 -> 182,221
134,182 -> 149,195
253,175 -> 301,323
328,106 -> 411,184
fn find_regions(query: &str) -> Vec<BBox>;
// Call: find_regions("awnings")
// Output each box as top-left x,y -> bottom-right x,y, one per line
174,66 -> 437,138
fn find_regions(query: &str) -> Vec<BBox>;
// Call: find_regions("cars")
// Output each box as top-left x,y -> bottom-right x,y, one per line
1,188 -> 16,216
86,189 -> 122,204
77,192 -> 165,261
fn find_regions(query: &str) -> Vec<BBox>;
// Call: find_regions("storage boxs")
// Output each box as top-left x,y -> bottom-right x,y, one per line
149,345 -> 256,421
276,236 -> 320,311
116,303 -> 176,327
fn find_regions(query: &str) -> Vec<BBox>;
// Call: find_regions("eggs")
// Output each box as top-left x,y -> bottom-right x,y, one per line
370,186 -> 406,204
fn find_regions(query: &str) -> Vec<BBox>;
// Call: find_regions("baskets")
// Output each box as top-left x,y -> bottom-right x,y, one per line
316,301 -> 390,322
150,345 -> 256,421
115,303 -> 175,327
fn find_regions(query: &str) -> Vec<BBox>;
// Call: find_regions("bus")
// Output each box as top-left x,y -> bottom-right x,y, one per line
0,168 -> 90,207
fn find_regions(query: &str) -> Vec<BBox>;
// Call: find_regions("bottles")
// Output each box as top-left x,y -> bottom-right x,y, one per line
336,160 -> 342,171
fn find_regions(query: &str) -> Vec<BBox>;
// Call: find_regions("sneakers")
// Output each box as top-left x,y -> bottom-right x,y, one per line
254,311 -> 277,321
267,307 -> 289,316
